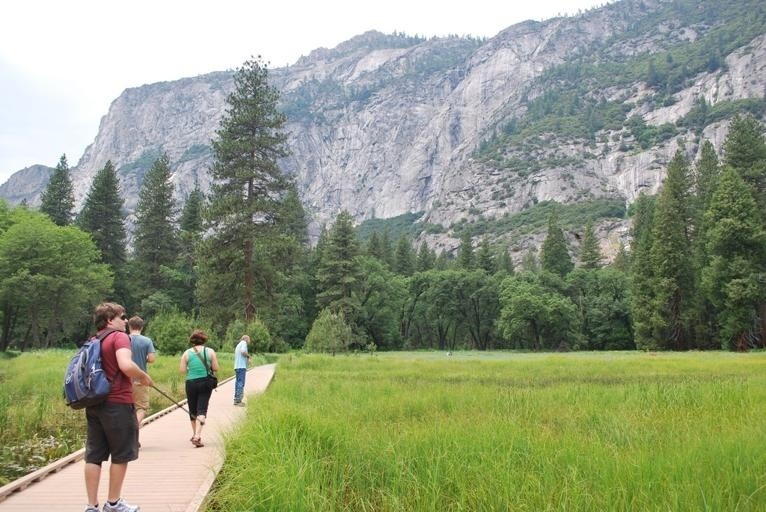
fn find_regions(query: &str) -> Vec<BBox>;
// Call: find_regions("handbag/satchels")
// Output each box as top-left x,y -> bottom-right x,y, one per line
206,374 -> 218,389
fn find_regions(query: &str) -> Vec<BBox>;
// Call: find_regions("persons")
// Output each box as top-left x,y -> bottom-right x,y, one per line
180,330 -> 219,446
127,315 -> 156,447
84,302 -> 155,512
233,334 -> 252,407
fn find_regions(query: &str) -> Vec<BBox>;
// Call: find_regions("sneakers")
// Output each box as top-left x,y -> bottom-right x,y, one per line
193,437 -> 204,447
233,401 -> 245,407
86,498 -> 140,512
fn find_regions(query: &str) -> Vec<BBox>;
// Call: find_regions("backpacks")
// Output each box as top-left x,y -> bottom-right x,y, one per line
62,327 -> 132,411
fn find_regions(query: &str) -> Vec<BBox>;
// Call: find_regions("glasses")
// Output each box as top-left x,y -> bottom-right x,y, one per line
120,315 -> 128,320
